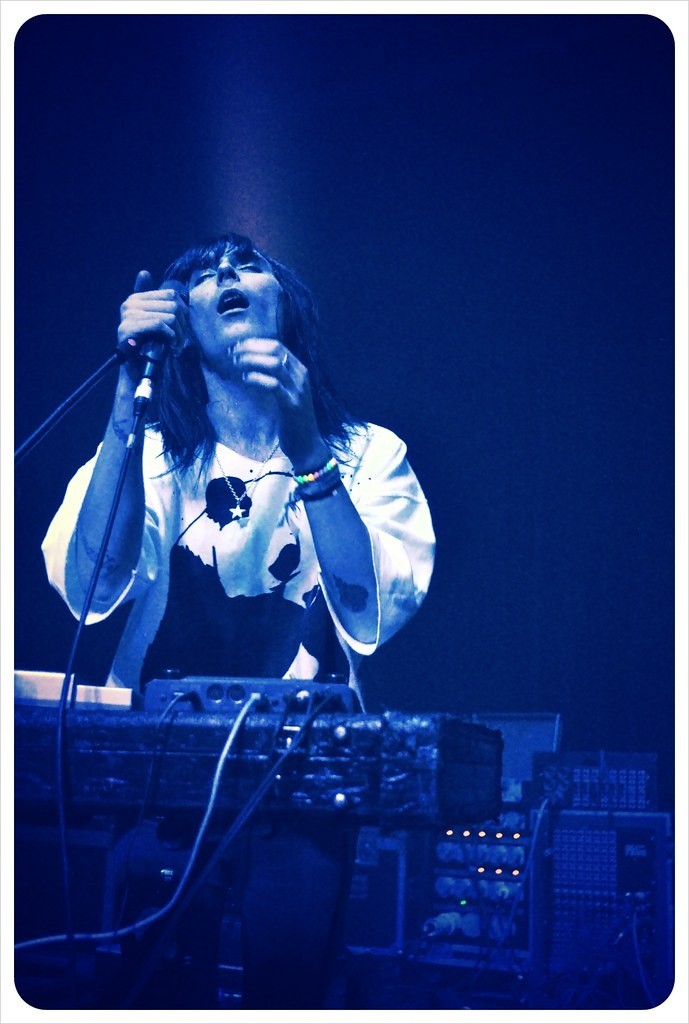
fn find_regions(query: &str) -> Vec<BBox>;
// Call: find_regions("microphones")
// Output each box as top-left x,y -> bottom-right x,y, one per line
133,278 -> 190,418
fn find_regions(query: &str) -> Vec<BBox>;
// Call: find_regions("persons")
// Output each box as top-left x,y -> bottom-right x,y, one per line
40,230 -> 439,1008
